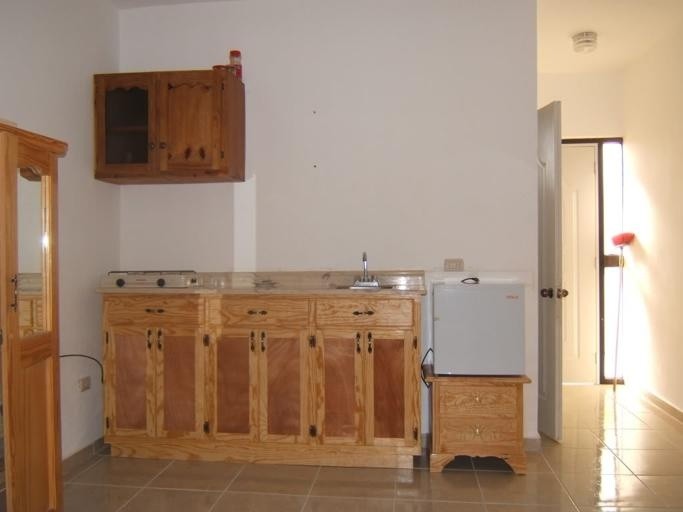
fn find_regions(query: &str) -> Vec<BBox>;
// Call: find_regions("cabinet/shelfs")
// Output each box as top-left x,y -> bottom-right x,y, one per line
204,295 -> 313,465
313,295 -> 421,469
423,364 -> 531,475
0,123 -> 68,512
93,69 -> 245,183
104,294 -> 203,461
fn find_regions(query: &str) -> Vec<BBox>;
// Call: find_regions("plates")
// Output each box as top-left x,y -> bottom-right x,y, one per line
251,280 -> 277,286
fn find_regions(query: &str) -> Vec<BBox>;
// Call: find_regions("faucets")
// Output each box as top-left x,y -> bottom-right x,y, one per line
361,252 -> 368,276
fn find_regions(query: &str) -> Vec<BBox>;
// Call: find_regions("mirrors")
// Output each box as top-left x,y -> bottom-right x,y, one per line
17,167 -> 46,337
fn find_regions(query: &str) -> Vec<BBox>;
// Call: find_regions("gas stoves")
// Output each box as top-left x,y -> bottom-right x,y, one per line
106,270 -> 196,288
